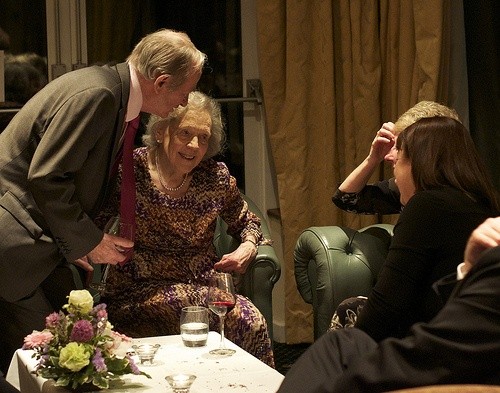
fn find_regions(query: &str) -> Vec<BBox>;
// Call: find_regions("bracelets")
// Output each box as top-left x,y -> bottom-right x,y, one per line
244,239 -> 258,250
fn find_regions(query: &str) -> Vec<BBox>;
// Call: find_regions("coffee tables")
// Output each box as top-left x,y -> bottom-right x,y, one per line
4,329 -> 286,393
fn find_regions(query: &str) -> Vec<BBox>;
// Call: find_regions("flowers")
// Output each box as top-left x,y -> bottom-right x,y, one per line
24,289 -> 153,393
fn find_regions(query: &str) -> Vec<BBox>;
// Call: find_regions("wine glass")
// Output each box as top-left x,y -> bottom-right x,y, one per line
208,273 -> 236,356
89,216 -> 133,293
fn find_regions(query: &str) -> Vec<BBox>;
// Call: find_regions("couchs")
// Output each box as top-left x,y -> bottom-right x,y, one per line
211,194 -> 281,349
293,222 -> 395,351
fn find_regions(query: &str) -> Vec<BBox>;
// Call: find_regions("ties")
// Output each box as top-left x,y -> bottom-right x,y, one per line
116,114 -> 140,267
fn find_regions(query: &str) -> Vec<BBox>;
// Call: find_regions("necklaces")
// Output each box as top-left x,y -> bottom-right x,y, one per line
154,154 -> 189,191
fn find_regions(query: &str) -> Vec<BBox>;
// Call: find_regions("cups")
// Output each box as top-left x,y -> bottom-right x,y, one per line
179,306 -> 209,347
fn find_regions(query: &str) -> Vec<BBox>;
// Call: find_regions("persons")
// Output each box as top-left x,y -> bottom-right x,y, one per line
326,100 -> 463,330
348,114 -> 500,344
72,89 -> 275,371
1,52 -> 48,132
1,27 -> 207,380
275,218 -> 500,393
